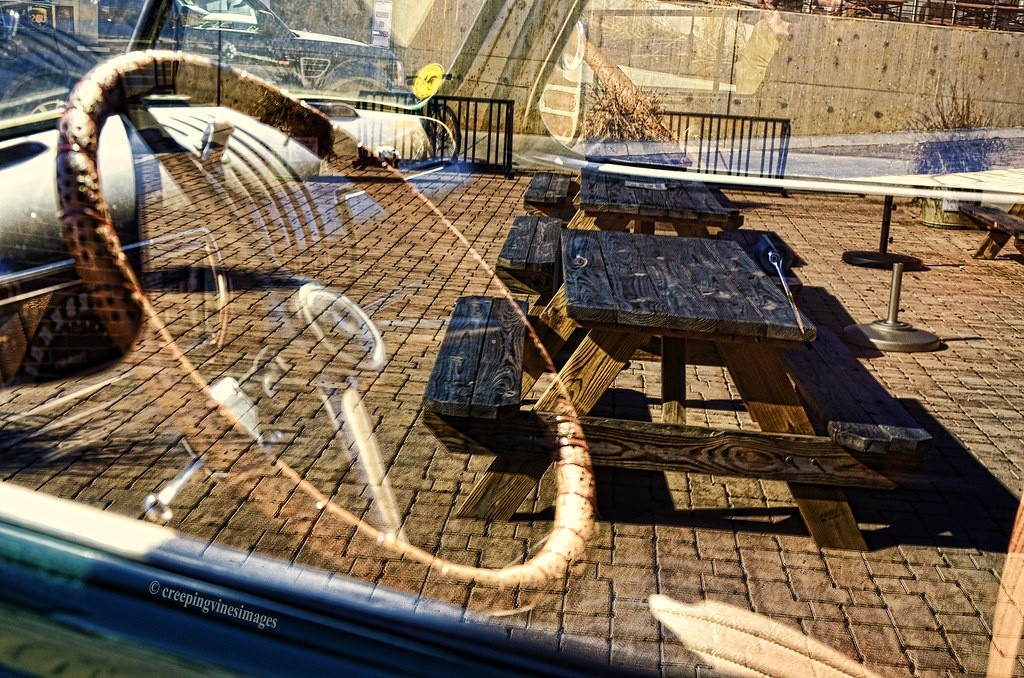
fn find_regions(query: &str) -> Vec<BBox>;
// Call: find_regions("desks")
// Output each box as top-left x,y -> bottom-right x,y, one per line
424,230 -> 939,553
497,167 -> 803,321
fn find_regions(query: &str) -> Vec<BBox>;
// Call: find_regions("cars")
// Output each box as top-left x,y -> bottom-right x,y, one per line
0,0 -> 406,132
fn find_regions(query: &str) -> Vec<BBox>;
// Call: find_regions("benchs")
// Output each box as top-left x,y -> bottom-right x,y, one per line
706,183 -> 743,228
524,172 -> 572,205
779,324 -> 933,455
959,205 -> 1024,257
423,296 -> 529,420
498,216 -> 563,272
719,229 -> 807,276
